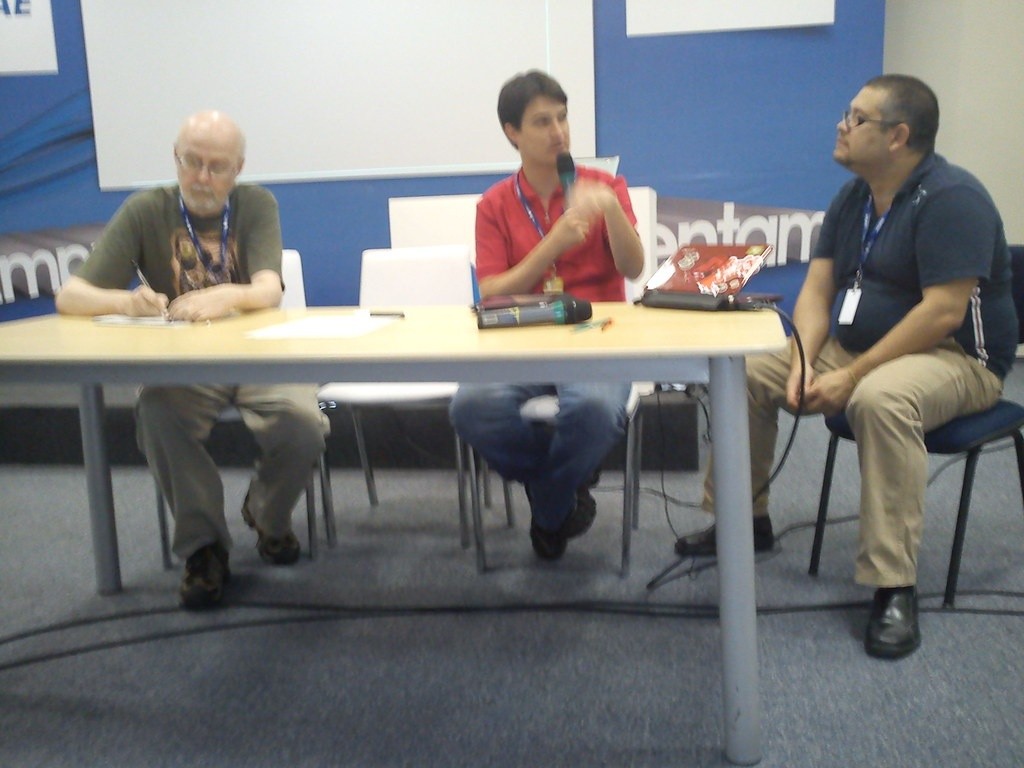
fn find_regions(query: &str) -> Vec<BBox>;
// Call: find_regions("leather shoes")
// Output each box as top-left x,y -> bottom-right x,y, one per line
865,585 -> 921,660
676,519 -> 774,556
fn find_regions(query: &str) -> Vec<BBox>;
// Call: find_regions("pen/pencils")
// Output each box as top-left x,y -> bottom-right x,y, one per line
130,259 -> 169,315
569,318 -> 611,334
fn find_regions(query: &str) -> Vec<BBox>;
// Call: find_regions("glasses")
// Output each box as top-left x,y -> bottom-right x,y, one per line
173,146 -> 240,179
840,109 -> 912,150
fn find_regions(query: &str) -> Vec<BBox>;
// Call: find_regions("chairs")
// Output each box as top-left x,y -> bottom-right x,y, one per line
807,245 -> 1024,607
151,187 -> 658,571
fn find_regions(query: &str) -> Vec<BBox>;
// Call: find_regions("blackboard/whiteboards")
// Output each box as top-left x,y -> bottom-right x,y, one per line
80,0 -> 595,191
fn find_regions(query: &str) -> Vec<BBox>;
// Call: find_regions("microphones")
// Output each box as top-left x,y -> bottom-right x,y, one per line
478,298 -> 593,330
555,151 -> 576,192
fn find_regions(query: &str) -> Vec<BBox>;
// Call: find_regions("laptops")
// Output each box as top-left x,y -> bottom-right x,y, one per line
640,243 -> 784,311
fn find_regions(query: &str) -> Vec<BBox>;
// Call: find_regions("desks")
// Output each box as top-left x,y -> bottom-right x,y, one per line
0,303 -> 787,768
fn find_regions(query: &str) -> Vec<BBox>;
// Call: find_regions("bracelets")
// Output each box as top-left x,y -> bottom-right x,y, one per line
848,366 -> 859,386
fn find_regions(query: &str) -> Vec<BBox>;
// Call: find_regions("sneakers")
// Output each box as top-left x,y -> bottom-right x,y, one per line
180,543 -> 229,607
240,490 -> 300,567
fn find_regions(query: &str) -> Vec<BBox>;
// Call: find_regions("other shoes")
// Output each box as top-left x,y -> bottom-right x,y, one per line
567,464 -> 600,539
523,483 -> 568,560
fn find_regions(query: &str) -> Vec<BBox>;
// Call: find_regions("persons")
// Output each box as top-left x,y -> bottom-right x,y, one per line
676,74 -> 1019,654
57,111 -> 329,609
449,72 -> 645,560
